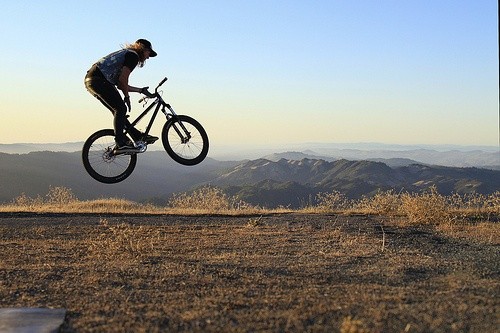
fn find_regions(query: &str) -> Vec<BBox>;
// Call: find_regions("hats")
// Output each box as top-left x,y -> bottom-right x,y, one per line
136,39 -> 157,57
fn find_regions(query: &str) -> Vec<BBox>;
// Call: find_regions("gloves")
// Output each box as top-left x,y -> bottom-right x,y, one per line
140,87 -> 152,98
123,96 -> 131,112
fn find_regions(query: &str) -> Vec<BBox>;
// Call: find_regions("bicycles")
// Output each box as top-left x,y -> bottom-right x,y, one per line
81,76 -> 210,185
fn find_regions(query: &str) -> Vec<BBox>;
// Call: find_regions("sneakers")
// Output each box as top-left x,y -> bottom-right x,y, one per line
136,132 -> 158,145
116,140 -> 144,152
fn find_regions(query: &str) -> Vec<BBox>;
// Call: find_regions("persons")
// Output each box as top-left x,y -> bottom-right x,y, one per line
84,39 -> 159,154
